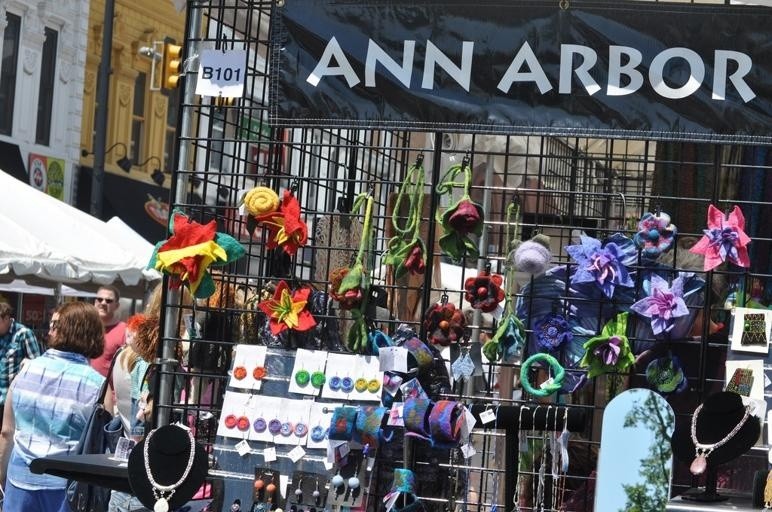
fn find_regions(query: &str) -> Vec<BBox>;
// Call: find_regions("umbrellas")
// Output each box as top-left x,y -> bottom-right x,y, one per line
0,168 -> 174,292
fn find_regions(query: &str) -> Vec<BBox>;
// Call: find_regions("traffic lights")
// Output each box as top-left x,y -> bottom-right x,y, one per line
159,36 -> 182,97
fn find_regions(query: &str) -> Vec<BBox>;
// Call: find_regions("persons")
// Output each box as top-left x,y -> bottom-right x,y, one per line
1,297 -> 42,432
171,268 -> 236,512
88,282 -> 131,405
1,300 -> 116,511
103,280 -> 190,511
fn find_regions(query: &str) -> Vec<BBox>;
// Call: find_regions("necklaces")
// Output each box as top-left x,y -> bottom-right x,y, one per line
450,401 -> 572,511
141,420 -> 197,511
688,400 -> 753,476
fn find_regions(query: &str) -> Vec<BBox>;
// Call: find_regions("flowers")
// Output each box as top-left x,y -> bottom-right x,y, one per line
148,155 -> 755,402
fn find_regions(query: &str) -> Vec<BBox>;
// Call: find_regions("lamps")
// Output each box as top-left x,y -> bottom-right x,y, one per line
79,141 -> 164,186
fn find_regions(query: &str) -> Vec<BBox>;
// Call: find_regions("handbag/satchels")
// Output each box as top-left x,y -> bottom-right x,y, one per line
65,404 -> 124,512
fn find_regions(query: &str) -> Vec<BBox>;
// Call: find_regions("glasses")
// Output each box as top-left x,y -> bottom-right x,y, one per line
96,298 -> 116,303
